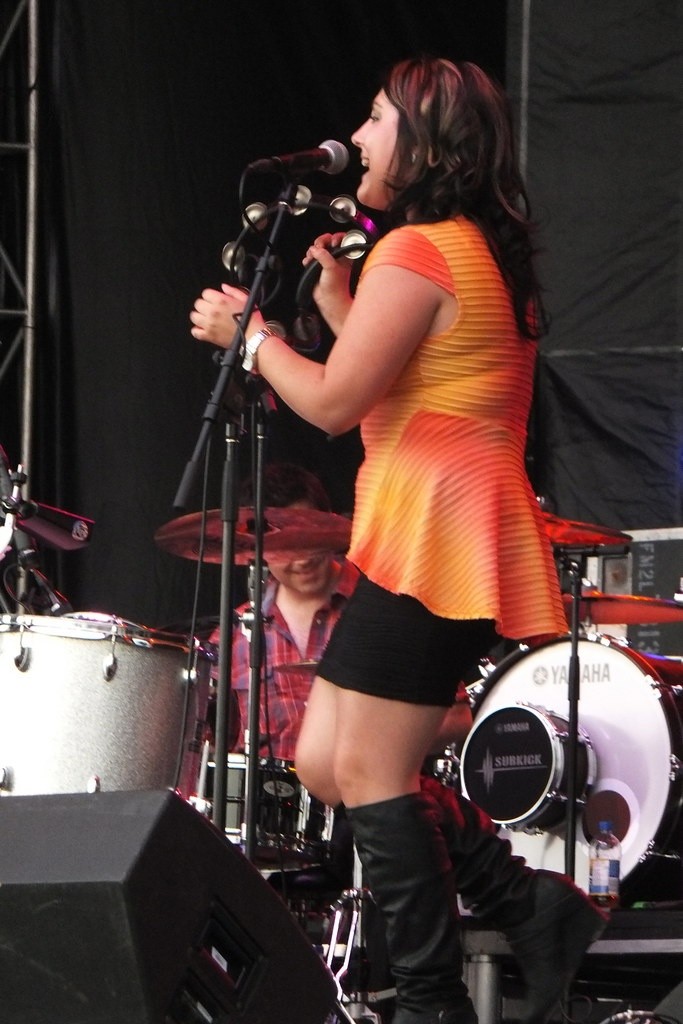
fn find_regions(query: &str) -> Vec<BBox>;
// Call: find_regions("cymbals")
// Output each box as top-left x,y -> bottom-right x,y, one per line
271,657 -> 320,676
540,510 -> 634,547
561,592 -> 683,624
154,506 -> 352,566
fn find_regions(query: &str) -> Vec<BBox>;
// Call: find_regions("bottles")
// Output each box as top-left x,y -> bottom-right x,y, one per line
588,820 -> 622,911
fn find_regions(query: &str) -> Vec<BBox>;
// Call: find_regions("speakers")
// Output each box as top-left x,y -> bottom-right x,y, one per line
0,791 -> 335,1024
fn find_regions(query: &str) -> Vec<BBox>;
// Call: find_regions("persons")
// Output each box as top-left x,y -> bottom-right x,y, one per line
200,463 -> 473,762
189,58 -> 607,1024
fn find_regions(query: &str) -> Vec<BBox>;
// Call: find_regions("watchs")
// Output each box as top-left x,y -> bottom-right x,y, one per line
240,328 -> 277,374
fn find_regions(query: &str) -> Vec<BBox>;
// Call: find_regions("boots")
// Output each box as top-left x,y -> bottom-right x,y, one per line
415,776 -> 607,1024
346,791 -> 480,1024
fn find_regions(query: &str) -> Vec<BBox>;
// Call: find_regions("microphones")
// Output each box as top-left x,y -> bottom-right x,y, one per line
246,140 -> 350,174
31,570 -> 74,617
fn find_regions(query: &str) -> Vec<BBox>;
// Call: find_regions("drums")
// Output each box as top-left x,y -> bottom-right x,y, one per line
196,752 -> 336,866
460,632 -> 683,910
0,613 -> 220,884
458,703 -> 598,836
